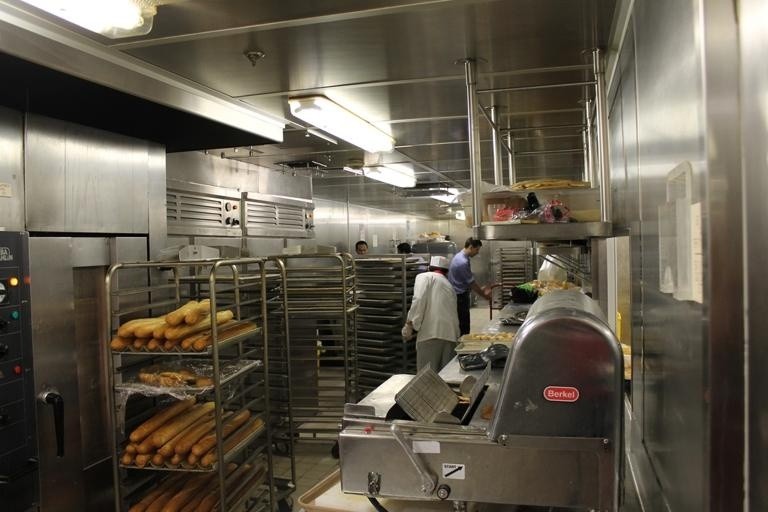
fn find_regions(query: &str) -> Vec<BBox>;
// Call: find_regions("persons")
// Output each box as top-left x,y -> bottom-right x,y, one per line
448,238 -> 492,336
397,243 -> 412,254
356,241 -> 368,255
401,256 -> 461,372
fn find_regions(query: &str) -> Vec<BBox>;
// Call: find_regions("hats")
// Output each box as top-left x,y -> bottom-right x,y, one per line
430,255 -> 450,270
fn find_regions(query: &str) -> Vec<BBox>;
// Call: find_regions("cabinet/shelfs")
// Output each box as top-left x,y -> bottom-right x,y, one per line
356,254 -> 429,402
104,256 -> 296,509
172,253 -> 358,458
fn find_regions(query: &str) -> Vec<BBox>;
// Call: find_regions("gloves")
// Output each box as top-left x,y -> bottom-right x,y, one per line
402,322 -> 414,341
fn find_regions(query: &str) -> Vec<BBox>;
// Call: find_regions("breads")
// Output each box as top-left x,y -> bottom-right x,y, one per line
515,279 -> 575,295
462,332 -> 516,342
481,403 -> 494,418
512,180 -> 575,190
108,297 -> 264,512
